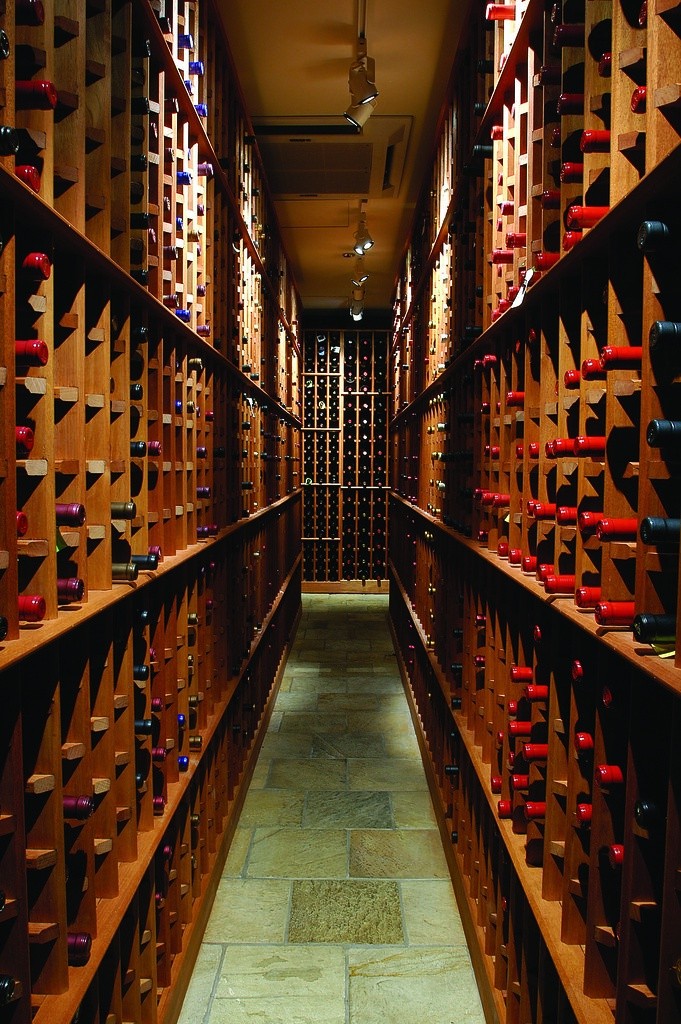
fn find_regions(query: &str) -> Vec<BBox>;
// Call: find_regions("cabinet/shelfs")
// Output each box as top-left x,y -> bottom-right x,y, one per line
0,0 -> 681,1024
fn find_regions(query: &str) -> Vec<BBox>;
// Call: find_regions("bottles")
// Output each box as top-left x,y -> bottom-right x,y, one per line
316,487 -> 326,538
306,334 -> 338,373
344,398 -> 385,487
303,540 -> 314,581
317,376 -> 327,428
390,0 -> 681,468
316,432 -> 326,483
390,468 -> 681,1024
329,486 -> 338,537
328,541 -> 338,581
305,376 -> 314,428
0,0 -> 303,474
315,540 -> 325,581
345,335 -> 386,393
304,486 -> 314,538
329,431 -> 339,482
344,491 -> 384,581
329,376 -> 339,427
304,432 -> 314,484
0,474 -> 303,1024
